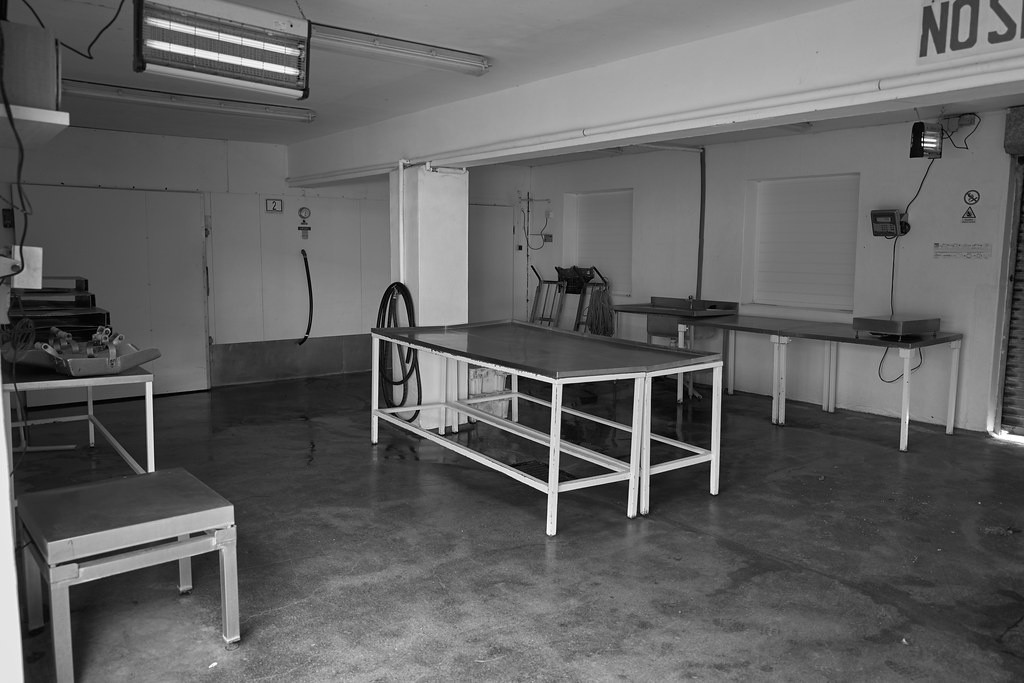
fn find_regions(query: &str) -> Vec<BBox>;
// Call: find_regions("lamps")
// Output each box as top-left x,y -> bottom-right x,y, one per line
133,1 -> 313,102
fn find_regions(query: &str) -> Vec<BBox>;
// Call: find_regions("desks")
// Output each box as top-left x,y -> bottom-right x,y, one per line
371,319 -> 725,537
1,355 -> 155,476
674,314 -> 963,453
16,464 -> 242,683
